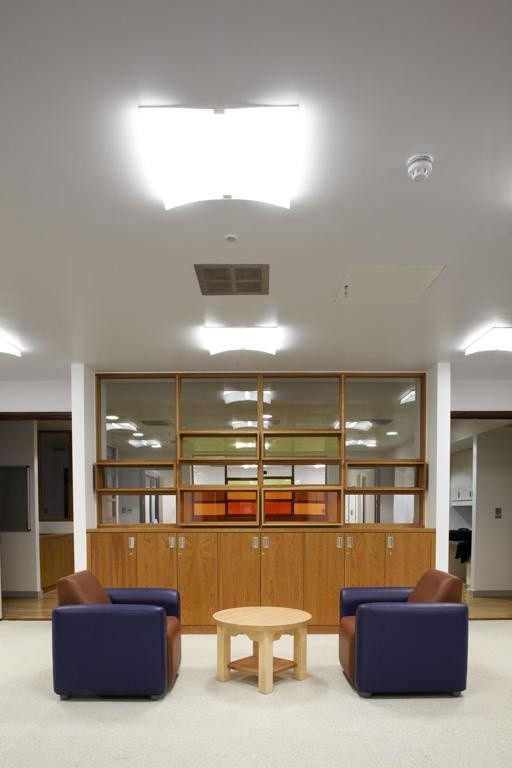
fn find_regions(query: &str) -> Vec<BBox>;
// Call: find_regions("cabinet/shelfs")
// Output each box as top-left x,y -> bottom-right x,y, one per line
89,373 -> 180,528
135,527 -> 218,632
183,375 -> 344,527
41,534 -> 74,592
386,526 -> 436,586
306,525 -> 385,635
345,371 -> 430,525
86,528 -> 135,589
220,527 -> 307,614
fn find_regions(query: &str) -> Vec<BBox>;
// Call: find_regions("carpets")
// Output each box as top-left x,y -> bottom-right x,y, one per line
1,633 -> 511,767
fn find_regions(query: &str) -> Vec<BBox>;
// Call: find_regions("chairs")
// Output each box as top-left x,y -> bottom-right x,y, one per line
49,569 -> 181,702
336,568 -> 468,698
